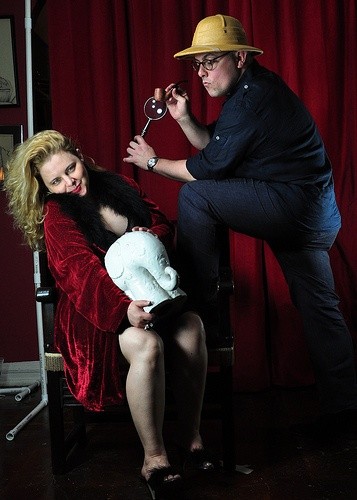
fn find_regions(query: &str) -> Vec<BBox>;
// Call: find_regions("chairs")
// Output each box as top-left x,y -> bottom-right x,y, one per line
33,225 -> 237,483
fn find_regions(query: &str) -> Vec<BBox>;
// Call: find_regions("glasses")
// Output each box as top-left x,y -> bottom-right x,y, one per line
192,52 -> 230,72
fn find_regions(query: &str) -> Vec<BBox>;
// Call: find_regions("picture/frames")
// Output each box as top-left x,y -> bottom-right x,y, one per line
0,14 -> 21,108
0,124 -> 24,192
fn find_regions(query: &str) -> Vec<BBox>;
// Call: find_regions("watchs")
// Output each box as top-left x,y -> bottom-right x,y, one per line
147,157 -> 161,172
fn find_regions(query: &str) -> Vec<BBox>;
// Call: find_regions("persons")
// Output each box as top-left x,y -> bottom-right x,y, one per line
124,14 -> 357,456
5,129 -> 225,494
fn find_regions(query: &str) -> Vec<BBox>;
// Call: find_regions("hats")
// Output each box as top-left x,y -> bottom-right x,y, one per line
173,14 -> 263,60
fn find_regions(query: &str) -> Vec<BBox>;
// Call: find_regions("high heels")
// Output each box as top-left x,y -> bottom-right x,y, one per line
184,446 -> 221,472
140,465 -> 188,499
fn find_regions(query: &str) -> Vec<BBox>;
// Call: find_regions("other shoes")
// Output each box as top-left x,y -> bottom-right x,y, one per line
181,288 -> 219,315
308,410 -> 357,438
